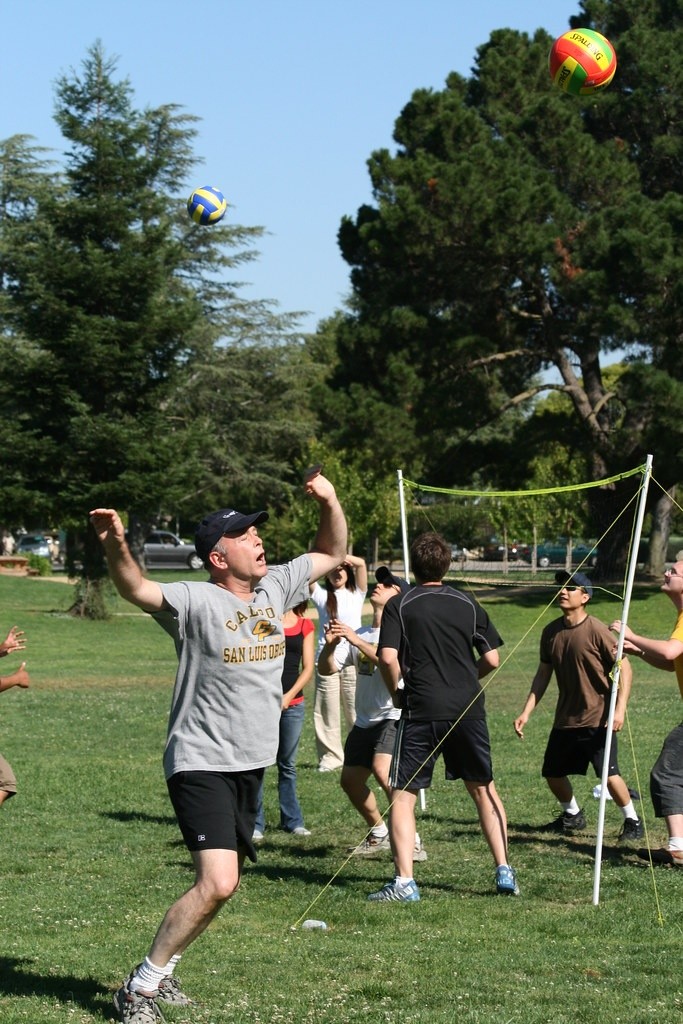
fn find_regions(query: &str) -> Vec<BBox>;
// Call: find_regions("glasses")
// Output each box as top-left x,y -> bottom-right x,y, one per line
665,568 -> 683,578
559,583 -> 586,593
379,577 -> 400,594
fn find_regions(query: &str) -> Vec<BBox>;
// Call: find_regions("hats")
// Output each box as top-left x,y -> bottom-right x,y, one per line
555,570 -> 593,597
195,509 -> 269,562
375,566 -> 411,594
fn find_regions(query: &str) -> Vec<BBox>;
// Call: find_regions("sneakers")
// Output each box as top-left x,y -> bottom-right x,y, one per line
496,865 -> 520,896
114,978 -> 157,1024
347,829 -> 392,855
618,816 -> 644,842
637,848 -> 683,867
368,875 -> 420,902
251,829 -> 264,843
544,810 -> 586,834
412,839 -> 427,862
292,827 -> 311,835
122,962 -> 196,1008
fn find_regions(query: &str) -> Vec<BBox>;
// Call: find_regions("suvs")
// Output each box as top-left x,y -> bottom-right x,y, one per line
125,528 -> 205,571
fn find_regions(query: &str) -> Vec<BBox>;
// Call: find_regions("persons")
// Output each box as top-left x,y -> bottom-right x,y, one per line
310,554 -> 368,771
318,567 -> 427,862
513,571 -> 644,839
0,625 -> 29,805
89,465 -> 346,1024
2,531 -> 15,554
368,535 -> 518,903
252,601 -> 315,839
609,550 -> 683,864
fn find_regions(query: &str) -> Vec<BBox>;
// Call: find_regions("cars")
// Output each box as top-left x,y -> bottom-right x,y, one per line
13,533 -> 53,565
478,535 -> 519,561
449,544 -> 469,563
523,537 -> 598,568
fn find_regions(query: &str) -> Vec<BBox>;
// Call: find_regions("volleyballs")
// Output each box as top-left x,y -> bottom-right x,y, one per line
548,26 -> 618,98
186,186 -> 227,226
